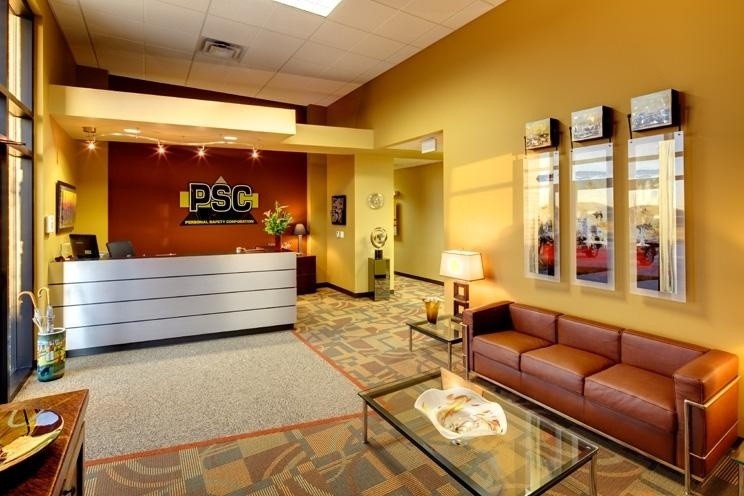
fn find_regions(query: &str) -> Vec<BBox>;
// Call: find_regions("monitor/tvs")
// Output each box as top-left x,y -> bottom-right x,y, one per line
106,240 -> 135,259
69,233 -> 100,260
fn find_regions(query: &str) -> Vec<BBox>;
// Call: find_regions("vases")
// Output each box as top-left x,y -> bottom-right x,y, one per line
420,297 -> 442,326
274,234 -> 283,251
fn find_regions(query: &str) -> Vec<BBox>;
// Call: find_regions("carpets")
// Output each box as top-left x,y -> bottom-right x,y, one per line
10,329 -> 394,464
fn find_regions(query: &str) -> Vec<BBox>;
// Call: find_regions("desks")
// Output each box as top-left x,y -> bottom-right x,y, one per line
1,389 -> 90,496
256,245 -> 316,296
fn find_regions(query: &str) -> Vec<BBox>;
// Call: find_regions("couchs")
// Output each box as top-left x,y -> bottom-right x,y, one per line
461,300 -> 740,496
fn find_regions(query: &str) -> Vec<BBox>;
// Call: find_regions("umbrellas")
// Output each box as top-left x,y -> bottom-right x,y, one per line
37,287 -> 57,332
18,291 -> 45,332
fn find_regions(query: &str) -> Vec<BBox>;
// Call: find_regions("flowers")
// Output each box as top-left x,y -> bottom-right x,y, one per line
264,201 -> 292,234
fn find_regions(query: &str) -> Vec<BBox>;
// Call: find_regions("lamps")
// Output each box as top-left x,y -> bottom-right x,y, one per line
439,249 -> 484,325
294,223 -> 309,255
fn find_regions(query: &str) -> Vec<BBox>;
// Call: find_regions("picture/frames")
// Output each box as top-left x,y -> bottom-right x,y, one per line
331,195 -> 346,226
55,181 -> 79,233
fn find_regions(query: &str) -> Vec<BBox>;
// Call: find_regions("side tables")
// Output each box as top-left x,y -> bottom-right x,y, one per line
404,313 -> 463,372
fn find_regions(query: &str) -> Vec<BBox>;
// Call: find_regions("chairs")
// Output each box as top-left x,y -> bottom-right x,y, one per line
107,238 -> 136,258
